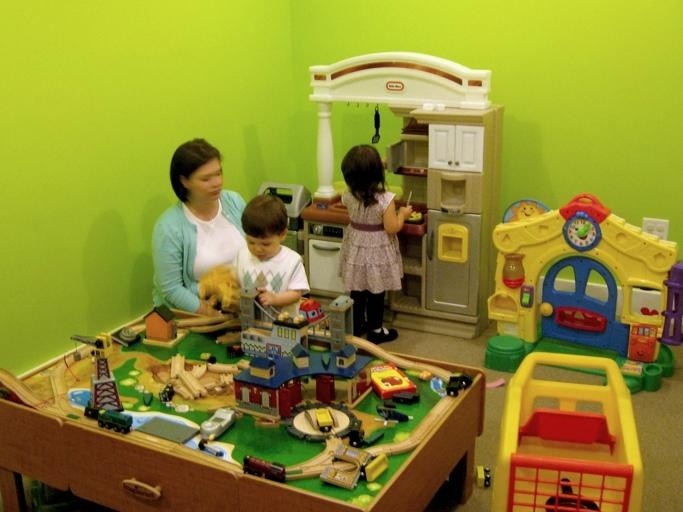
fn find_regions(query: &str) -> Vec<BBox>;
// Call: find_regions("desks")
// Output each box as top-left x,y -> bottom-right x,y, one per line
0,306 -> 486,510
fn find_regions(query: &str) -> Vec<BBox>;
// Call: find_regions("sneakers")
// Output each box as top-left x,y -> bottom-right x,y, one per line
366,326 -> 399,345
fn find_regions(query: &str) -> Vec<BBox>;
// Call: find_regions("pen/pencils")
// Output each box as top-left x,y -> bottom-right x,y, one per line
406,191 -> 412,207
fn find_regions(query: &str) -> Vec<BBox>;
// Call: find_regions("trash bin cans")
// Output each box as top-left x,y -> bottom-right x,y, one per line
257,181 -> 312,257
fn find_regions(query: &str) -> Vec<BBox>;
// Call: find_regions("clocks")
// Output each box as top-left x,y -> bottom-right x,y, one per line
561,210 -> 602,252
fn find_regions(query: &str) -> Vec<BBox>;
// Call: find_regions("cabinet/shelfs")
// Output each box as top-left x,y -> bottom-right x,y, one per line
425,106 -> 503,316
385,232 -> 424,314
385,129 -> 427,177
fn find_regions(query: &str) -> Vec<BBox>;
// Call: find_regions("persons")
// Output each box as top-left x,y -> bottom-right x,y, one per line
338,145 -> 414,345
232,195 -> 311,320
151,140 -> 248,316
